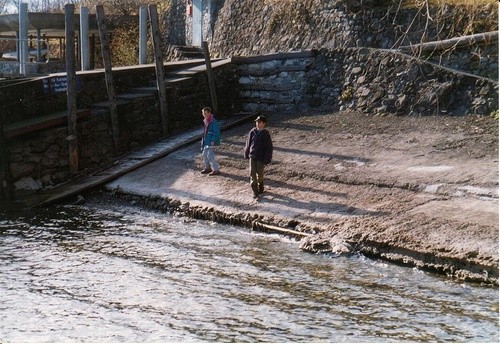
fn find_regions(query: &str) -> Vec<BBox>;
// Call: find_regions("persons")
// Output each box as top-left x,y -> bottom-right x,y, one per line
244,115 -> 273,200
201,107 -> 222,176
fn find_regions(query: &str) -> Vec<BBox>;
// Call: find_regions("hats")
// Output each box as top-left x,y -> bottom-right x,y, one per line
254,116 -> 266,122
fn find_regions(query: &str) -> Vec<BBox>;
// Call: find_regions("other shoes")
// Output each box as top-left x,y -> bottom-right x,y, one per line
258,191 -> 264,194
209,171 -> 220,175
201,168 -> 212,174
252,194 -> 258,199
